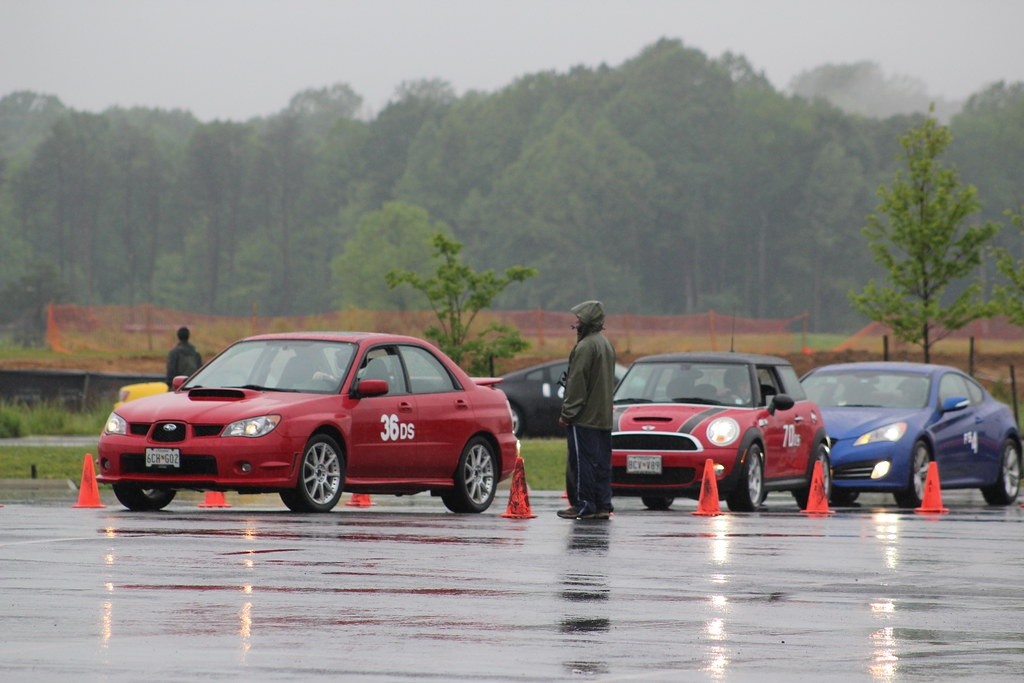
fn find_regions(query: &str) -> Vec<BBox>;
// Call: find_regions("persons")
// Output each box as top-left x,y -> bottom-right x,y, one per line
313,343 -> 370,398
557,299 -> 614,520
165,326 -> 204,392
722,371 -> 762,409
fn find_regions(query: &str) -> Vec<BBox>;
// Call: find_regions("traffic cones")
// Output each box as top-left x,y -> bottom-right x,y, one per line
71,452 -> 108,509
560,490 -> 569,499
912,461 -> 950,513
689,459 -> 725,515
798,460 -> 836,514
500,456 -> 538,519
196,490 -> 233,508
345,493 -> 376,507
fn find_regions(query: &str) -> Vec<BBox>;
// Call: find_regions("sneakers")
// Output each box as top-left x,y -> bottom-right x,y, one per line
556,507 -> 595,518
595,509 -> 609,518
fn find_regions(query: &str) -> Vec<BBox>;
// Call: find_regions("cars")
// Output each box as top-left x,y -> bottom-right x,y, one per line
597,351 -> 828,513
96,328 -> 520,512
793,361 -> 1023,509
482,354 -> 650,439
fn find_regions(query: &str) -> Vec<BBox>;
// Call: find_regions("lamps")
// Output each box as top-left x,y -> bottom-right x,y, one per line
368,358 -> 392,393
275,357 -> 325,389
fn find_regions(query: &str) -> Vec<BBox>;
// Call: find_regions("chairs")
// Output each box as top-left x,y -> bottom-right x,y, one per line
757,385 -> 779,405
694,384 -> 718,401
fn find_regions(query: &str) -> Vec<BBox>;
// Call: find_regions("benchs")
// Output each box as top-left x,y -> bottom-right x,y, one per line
411,378 -> 445,395
